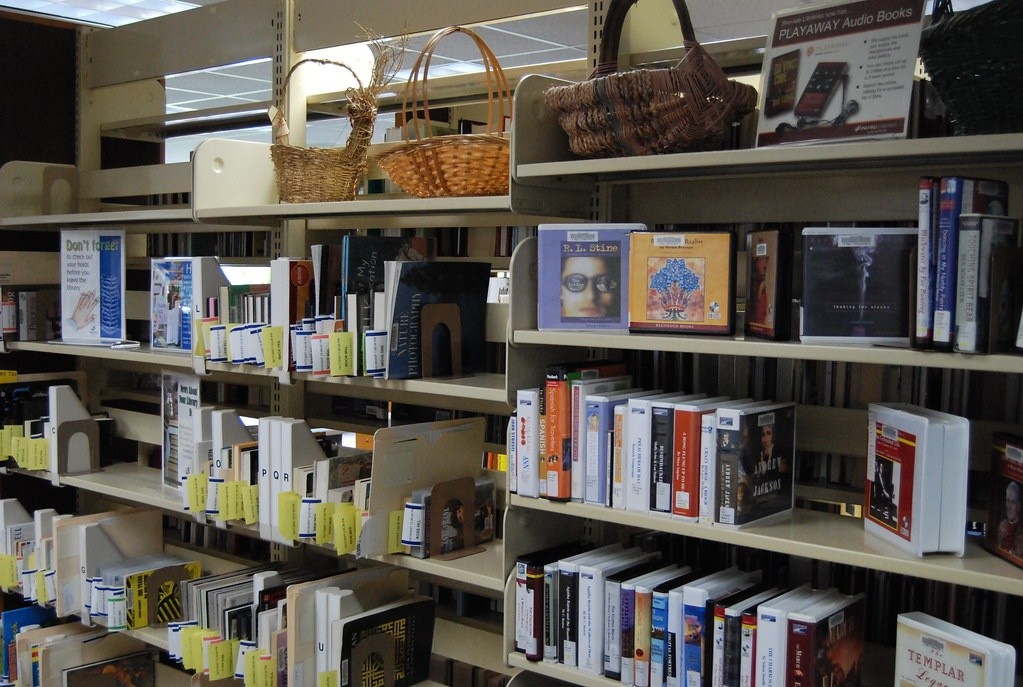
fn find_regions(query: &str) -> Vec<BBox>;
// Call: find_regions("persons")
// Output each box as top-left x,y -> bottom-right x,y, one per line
748,422 -> 784,505
560,255 -> 621,319
65,289 -> 100,331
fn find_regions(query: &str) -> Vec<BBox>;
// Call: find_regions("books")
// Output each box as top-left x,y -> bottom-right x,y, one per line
24,410 -> 116,469
195,430 -> 412,556
915,176 -> 1023,355
61,650 -> 156,687
123,560 -> 435,686
310,233 -> 492,381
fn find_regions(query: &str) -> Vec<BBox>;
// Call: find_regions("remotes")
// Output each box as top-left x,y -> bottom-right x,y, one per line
793,60 -> 848,119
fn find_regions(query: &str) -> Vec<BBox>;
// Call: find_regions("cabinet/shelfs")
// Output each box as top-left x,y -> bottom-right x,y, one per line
0,73 -> 1023,687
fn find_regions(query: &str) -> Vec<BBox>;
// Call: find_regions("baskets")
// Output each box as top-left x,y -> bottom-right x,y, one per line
545,0 -> 759,158
270,23 -> 408,203
374,25 -> 512,197
920,0 -> 1023,135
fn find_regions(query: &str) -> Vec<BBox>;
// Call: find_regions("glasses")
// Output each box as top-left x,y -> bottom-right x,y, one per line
562,274 -> 617,292
